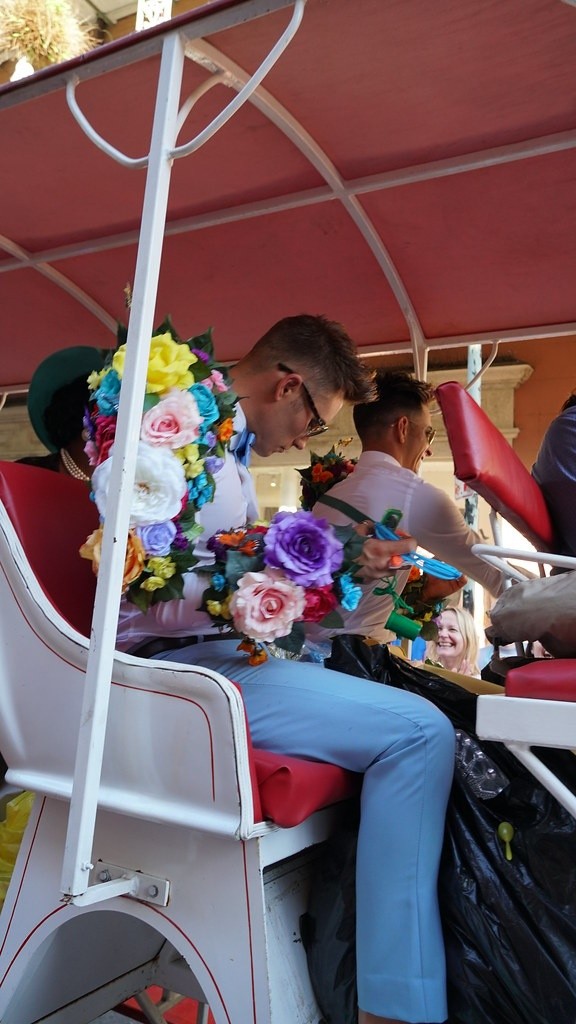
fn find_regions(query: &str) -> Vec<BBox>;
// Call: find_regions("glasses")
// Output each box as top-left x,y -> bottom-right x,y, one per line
278,362 -> 329,437
391,419 -> 437,444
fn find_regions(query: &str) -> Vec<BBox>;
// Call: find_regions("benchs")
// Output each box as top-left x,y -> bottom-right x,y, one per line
0,460 -> 367,869
436,381 -> 576,751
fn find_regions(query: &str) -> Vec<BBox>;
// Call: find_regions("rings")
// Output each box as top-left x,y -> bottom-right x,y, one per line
361,521 -> 370,528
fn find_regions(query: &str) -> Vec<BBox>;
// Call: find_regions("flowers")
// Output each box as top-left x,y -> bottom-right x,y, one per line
78,319 -> 452,665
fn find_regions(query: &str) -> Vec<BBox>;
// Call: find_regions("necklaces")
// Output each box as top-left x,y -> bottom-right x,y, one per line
60,448 -> 90,481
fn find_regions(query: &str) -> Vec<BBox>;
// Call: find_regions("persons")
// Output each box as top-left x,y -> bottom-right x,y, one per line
113,309 -> 458,1024
10,345 -> 118,482
307,372 -> 536,813
424,605 -> 482,680
529,388 -> 576,575
526,640 -> 554,659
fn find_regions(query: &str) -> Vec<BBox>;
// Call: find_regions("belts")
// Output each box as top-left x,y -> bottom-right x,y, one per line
131,632 -> 243,659
